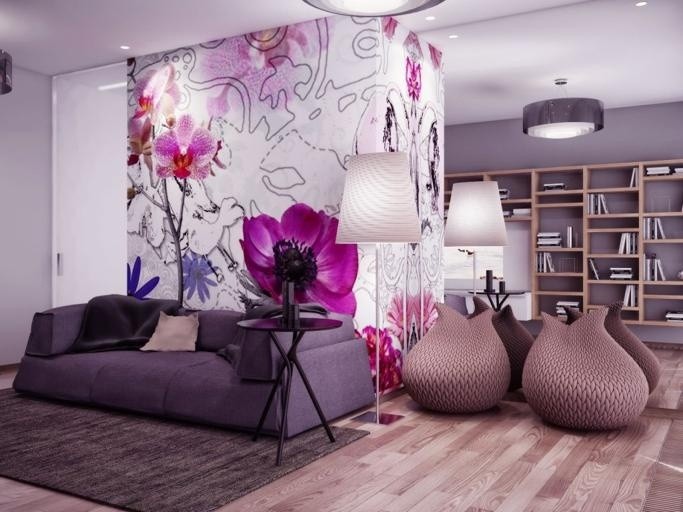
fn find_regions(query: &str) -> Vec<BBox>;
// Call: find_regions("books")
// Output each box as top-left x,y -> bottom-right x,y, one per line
566,224 -> 573,248
544,182 -> 565,191
618,232 -> 636,255
499,188 -> 510,200
502,209 -> 511,216
644,217 -> 665,240
609,267 -> 633,280
537,232 -> 562,247
673,168 -> 683,175
644,252 -> 665,282
646,167 -> 670,176
555,301 -> 580,318
513,208 -> 531,215
623,285 -> 636,307
590,258 -> 600,280
630,167 -> 639,187
665,311 -> 683,322
587,193 -> 608,215
536,252 -> 554,273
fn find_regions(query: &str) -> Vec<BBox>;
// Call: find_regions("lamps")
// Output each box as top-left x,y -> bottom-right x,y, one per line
443,182 -> 510,296
523,98 -> 604,139
335,152 -> 423,426
302,0 -> 443,17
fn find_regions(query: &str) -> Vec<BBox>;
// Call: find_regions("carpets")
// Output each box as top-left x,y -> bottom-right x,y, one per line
0,388 -> 370,512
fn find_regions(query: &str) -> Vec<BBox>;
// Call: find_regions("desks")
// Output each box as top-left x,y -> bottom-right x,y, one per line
238,318 -> 343,466
468,292 -> 526,312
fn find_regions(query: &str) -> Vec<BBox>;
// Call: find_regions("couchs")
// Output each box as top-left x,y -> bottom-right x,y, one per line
12,294 -> 376,440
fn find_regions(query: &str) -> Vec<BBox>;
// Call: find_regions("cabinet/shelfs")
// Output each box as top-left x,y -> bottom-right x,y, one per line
444,159 -> 683,327
